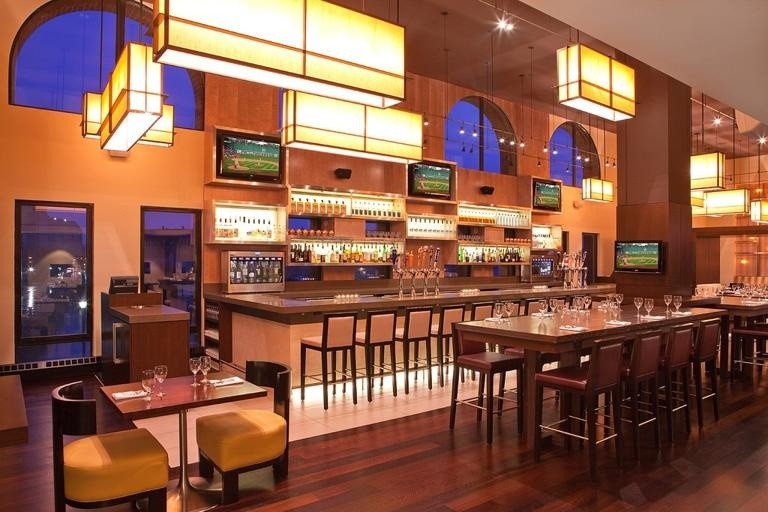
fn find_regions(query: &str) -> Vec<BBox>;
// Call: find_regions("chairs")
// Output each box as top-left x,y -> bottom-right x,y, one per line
50,381 -> 169,512
197,360 -> 292,507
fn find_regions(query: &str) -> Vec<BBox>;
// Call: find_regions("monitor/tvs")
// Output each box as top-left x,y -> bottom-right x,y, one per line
614,240 -> 664,273
533,180 -> 561,211
216,128 -> 284,184
408,161 -> 453,199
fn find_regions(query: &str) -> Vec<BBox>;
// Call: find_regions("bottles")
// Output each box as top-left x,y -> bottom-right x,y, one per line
532,234 -> 560,249
214,216 -> 275,241
290,196 -> 347,215
290,243 -> 400,263
459,209 -> 529,226
409,218 -> 455,237
351,201 -> 402,217
458,245 -> 525,263
532,258 -> 554,275
229,257 -> 282,283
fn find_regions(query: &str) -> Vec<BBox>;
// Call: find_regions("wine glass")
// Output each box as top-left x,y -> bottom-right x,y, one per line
690,282 -> 767,304
140,364 -> 170,402
634,297 -> 654,319
188,355 -> 212,386
602,294 -> 623,312
664,294 -> 683,315
495,302 -> 515,322
539,295 -> 591,327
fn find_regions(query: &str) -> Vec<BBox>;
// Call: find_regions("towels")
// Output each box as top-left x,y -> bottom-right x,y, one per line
209,377 -> 243,387
112,390 -> 147,401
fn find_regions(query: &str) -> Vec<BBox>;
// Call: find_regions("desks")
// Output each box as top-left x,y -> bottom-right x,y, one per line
27,298 -> 71,357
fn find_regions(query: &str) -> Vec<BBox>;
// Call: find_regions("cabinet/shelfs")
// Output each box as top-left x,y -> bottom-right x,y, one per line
101,292 -> 191,384
158,279 -> 201,359
202,125 -> 564,298
205,298 -> 232,371
